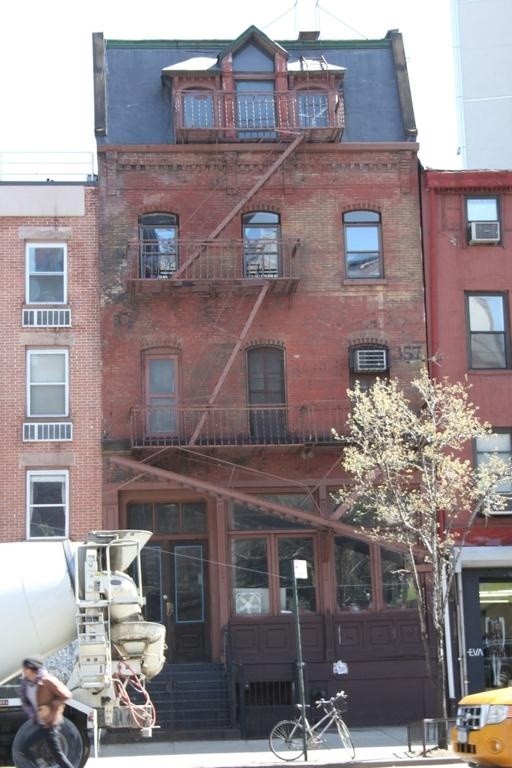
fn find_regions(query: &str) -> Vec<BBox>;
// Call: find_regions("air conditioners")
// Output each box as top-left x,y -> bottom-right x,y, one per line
467,218 -> 501,246
354,348 -> 388,373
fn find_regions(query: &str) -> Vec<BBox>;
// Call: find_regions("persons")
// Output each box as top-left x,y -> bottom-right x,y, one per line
19,659 -> 74,768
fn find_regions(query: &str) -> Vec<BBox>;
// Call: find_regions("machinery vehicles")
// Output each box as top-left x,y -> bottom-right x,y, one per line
0,525 -> 165,768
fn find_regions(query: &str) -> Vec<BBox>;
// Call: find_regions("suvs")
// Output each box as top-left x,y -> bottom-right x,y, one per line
450,684 -> 512,767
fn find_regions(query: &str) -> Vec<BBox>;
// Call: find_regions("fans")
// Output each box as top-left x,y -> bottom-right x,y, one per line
236,591 -> 263,616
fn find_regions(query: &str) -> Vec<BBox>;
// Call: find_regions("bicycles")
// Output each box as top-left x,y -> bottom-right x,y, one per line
268,688 -> 357,762
